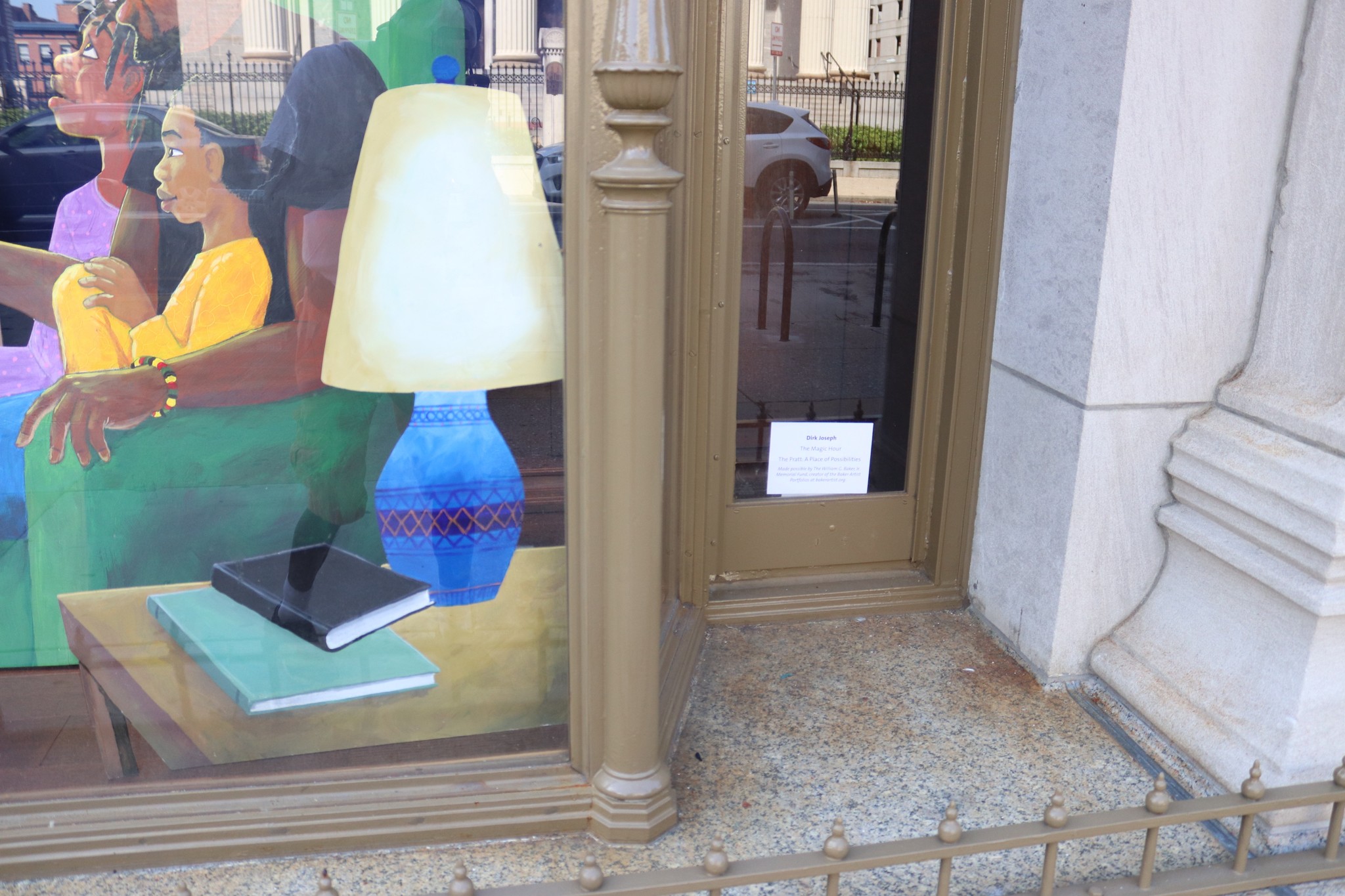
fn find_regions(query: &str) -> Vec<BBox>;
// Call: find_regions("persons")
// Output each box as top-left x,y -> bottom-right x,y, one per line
257,1 -> 487,645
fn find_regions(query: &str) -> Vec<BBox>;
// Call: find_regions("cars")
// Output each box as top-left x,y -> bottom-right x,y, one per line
1,104 -> 264,221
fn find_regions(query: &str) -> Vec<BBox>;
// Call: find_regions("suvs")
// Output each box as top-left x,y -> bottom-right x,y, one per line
535,102 -> 832,218
